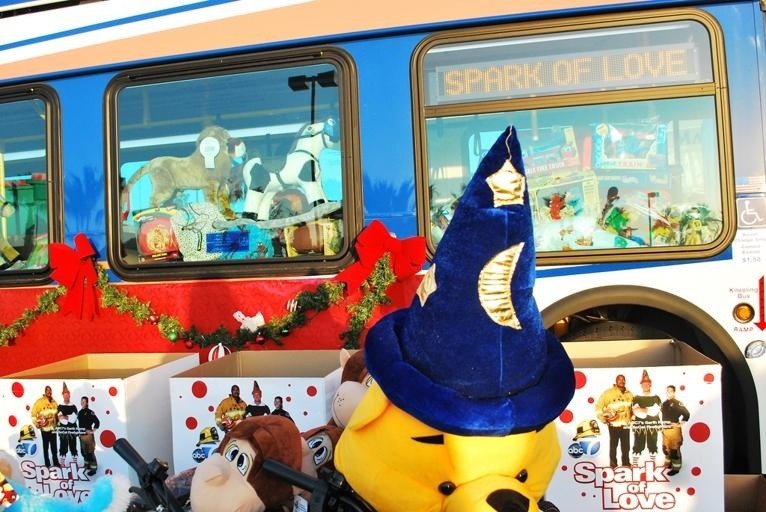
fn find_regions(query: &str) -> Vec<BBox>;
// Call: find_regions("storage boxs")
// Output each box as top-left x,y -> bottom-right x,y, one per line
169,350 -> 344,476
545,336 -> 726,510
0,348 -> 200,478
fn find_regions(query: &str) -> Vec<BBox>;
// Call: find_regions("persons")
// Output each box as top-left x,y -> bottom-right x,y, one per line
594,375 -> 634,469
56,392 -> 79,470
31,386 -> 60,469
271,395 -> 294,424
246,393 -> 270,417
77,396 -> 100,475
660,385 -> 689,476
214,385 -> 248,433
630,382 -> 661,469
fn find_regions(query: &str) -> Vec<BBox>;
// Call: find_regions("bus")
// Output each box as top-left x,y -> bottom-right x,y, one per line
0,0 -> 766,477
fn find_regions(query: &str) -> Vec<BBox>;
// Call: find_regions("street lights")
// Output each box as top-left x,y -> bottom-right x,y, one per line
287,69 -> 339,202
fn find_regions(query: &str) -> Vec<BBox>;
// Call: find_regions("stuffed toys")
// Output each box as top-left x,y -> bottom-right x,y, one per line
298,423 -> 344,502
331,126 -> 578,511
0,110 -> 728,271
327,349 -> 375,430
185,414 -> 302,510
122,117 -> 342,259
